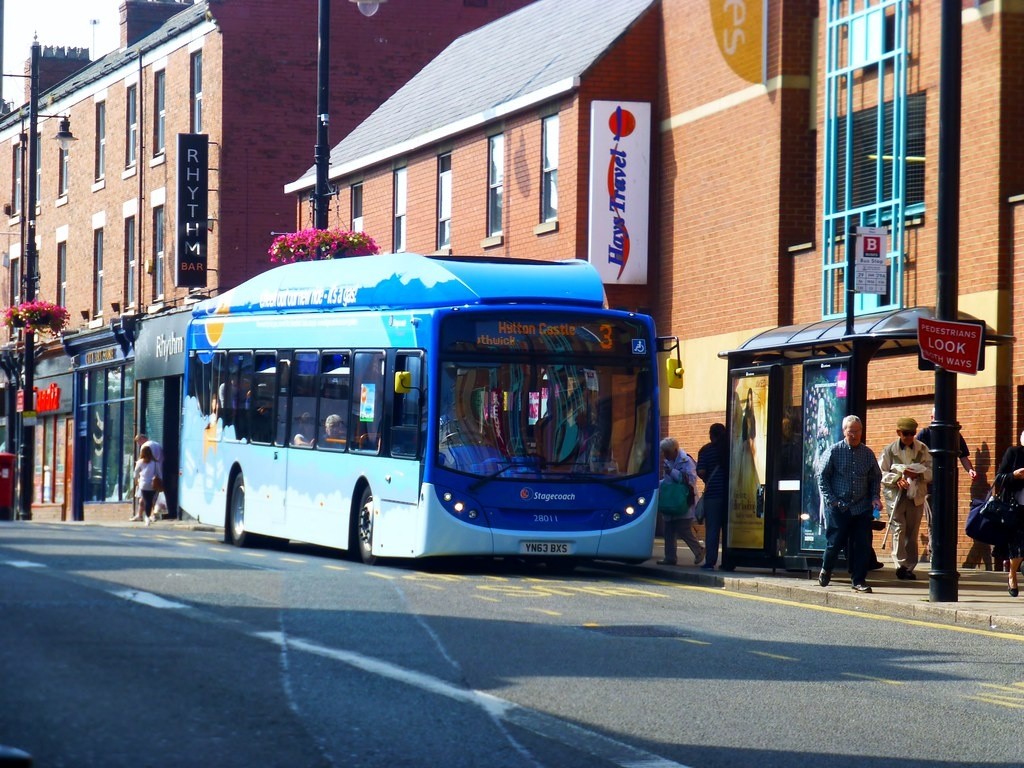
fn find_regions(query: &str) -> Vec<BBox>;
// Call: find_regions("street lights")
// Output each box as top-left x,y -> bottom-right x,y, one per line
15,30 -> 78,521
309,0 -> 382,262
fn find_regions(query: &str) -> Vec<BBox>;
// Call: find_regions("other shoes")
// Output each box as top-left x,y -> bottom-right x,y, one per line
895,567 -> 916,580
694,547 -> 705,565
1008,576 -> 1019,597
656,558 -> 677,566
718,564 -> 736,572
699,564 -> 714,572
129,513 -> 157,527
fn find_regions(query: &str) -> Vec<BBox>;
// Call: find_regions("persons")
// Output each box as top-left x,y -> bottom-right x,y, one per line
218,372 -> 238,408
696,423 -> 737,571
130,434 -> 163,527
319,414 -> 345,450
877,417 -> 932,580
916,407 -> 977,554
656,437 -> 705,565
992,428 -> 1024,597
731,388 -> 760,512
293,411 -> 315,446
818,415 -> 883,594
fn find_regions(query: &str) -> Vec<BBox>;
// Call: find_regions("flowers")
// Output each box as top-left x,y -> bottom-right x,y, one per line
0,299 -> 70,339
267,229 -> 382,264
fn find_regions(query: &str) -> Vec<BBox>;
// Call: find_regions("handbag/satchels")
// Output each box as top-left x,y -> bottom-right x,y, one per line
657,472 -> 695,516
980,474 -> 1019,526
153,490 -> 169,515
692,494 -> 705,526
964,474 -> 1011,546
151,475 -> 164,493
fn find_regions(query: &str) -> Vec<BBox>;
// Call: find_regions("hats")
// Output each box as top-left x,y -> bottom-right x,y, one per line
896,417 -> 918,431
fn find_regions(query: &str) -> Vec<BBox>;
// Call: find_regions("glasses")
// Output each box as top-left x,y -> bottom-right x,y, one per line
900,430 -> 917,437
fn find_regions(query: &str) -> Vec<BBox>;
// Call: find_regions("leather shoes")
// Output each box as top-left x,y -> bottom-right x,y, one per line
851,581 -> 872,593
818,568 -> 832,587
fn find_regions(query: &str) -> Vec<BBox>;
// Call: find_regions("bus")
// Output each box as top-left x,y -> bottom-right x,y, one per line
177,252 -> 688,567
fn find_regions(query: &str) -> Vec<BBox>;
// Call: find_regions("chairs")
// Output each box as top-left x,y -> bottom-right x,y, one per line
230,382 -> 272,440
